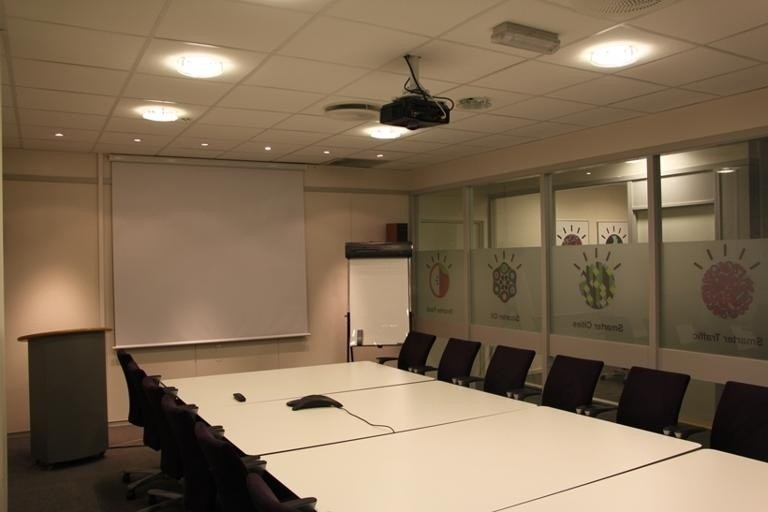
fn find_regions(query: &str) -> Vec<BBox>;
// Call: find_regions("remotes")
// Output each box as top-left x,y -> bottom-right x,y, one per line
233,392 -> 245,402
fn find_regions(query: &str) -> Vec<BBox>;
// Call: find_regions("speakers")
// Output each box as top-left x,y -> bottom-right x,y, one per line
386,222 -> 408,241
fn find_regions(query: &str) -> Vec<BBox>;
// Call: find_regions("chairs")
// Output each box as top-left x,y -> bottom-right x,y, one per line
136,375 -> 215,512
585,366 -> 692,435
376,330 -> 436,375
675,380 -> 768,463
112,347 -> 162,500
458,344 -> 537,397
415,335 -> 482,383
245,471 -> 316,512
514,353 -> 606,412
133,366 -> 176,452
194,421 -> 243,512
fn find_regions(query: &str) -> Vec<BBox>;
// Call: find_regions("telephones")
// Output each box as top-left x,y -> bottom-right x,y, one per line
286,396 -> 343,411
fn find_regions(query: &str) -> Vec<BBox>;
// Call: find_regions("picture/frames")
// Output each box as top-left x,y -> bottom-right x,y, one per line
555,219 -> 591,246
597,221 -> 628,245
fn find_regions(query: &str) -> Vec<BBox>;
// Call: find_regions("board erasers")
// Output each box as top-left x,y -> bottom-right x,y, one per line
357,330 -> 363,346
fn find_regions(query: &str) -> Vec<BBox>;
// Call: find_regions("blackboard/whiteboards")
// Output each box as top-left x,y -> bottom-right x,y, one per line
348,257 -> 410,346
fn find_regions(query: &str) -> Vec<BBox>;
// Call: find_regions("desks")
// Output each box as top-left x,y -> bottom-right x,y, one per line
192,380 -> 537,461
160,361 -> 437,410
493,449 -> 768,512
247,405 -> 703,511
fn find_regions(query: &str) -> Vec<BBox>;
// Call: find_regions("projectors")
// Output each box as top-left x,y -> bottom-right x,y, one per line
380,95 -> 452,133
